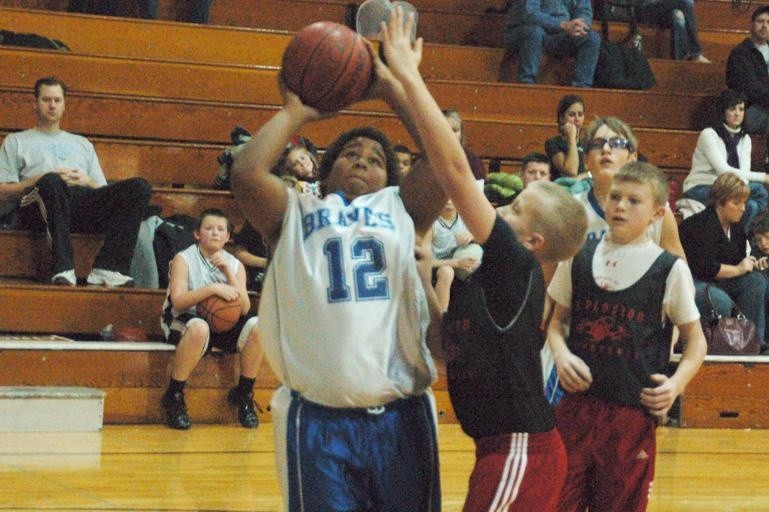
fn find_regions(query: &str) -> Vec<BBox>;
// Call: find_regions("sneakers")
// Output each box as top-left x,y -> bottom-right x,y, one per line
162,389 -> 189,429
52,269 -> 76,286
87,268 -> 133,287
228,387 -> 259,428
688,54 -> 712,63
521,75 -> 534,83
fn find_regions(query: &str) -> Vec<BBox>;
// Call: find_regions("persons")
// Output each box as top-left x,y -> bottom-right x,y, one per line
542,117 -> 707,510
381,7 -> 587,510
231,223 -> 271,288
0,77 -> 153,287
725,6 -> 769,132
159,207 -> 265,430
278,87 -> 769,344
228,38 -> 451,511
639,1 -> 712,62
504,1 -> 602,86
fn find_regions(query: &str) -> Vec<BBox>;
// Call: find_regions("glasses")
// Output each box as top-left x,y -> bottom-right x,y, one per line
586,136 -> 633,158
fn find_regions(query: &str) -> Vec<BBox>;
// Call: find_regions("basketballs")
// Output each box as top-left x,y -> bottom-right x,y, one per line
196,295 -> 242,335
282,21 -> 374,112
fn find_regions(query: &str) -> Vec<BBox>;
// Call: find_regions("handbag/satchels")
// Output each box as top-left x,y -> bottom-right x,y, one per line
703,315 -> 761,354
594,37 -> 656,89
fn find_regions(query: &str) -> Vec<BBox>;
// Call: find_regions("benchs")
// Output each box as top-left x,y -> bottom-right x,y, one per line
0,0 -> 768,433
